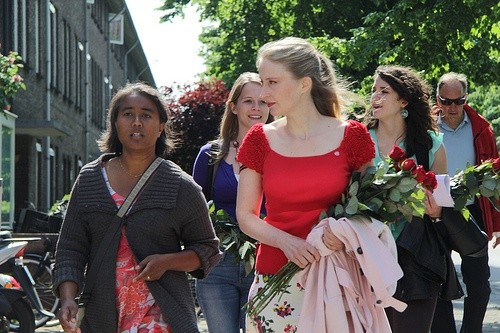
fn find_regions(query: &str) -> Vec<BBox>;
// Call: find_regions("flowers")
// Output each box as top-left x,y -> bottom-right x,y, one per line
240,145 -> 438,317
0,51 -> 26,120
448,157 -> 500,222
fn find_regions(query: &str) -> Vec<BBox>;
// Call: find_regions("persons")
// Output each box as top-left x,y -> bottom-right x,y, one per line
52,81 -> 225,333
192,71 -> 275,333
431,72 -> 500,333
235,36 -> 377,333
352,65 -> 447,333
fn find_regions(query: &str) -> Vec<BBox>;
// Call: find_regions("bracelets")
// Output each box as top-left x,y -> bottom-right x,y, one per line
429,217 -> 442,223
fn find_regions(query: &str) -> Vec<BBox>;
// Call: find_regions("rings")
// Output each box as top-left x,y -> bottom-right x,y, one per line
147,277 -> 151,281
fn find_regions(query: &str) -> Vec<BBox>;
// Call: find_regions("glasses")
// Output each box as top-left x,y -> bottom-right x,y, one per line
439,95 -> 466,105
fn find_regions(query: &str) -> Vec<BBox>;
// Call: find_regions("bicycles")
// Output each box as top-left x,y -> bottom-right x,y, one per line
0,236 -> 85,331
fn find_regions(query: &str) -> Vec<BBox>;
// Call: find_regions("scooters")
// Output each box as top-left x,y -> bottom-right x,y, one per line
0,230 -> 56,333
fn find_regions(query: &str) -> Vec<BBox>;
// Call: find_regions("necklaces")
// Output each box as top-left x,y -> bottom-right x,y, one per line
118,158 -> 142,178
